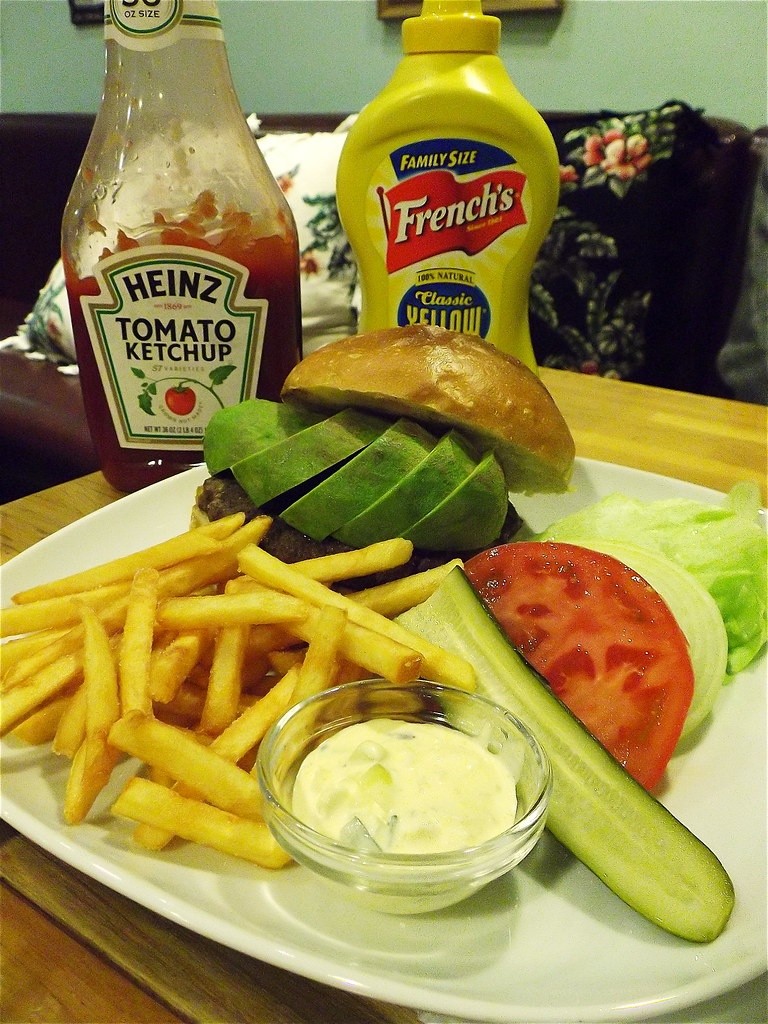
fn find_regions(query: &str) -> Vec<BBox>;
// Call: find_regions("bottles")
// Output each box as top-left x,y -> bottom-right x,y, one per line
59,0 -> 307,491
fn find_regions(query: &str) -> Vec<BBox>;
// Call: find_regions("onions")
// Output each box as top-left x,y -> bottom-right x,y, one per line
539,541 -> 728,746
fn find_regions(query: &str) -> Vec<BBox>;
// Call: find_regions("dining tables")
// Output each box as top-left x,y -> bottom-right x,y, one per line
0,362 -> 768,1024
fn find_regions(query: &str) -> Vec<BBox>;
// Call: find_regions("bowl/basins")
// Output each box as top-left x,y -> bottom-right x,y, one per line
257,672 -> 553,911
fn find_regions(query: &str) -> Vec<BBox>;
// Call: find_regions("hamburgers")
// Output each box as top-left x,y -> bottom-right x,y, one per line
183,325 -> 571,592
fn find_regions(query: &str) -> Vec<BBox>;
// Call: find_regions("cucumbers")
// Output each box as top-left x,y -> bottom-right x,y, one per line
378,565 -> 740,948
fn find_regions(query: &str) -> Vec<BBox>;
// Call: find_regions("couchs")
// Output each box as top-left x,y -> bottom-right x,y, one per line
2,112 -> 767,504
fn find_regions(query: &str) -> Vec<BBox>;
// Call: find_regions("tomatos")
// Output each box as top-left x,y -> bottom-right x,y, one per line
457,537 -> 691,801
162,386 -> 197,415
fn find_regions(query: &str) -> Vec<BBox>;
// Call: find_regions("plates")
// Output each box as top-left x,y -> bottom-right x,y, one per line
2,433 -> 768,1023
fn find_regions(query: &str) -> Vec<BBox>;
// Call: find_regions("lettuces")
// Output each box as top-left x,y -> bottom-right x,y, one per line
525,478 -> 767,686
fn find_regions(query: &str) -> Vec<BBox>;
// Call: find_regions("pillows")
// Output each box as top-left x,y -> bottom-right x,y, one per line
1,111 -> 372,381
526,99 -> 720,394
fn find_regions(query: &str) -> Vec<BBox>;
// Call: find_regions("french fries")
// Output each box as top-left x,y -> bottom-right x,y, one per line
1,508 -> 474,863
